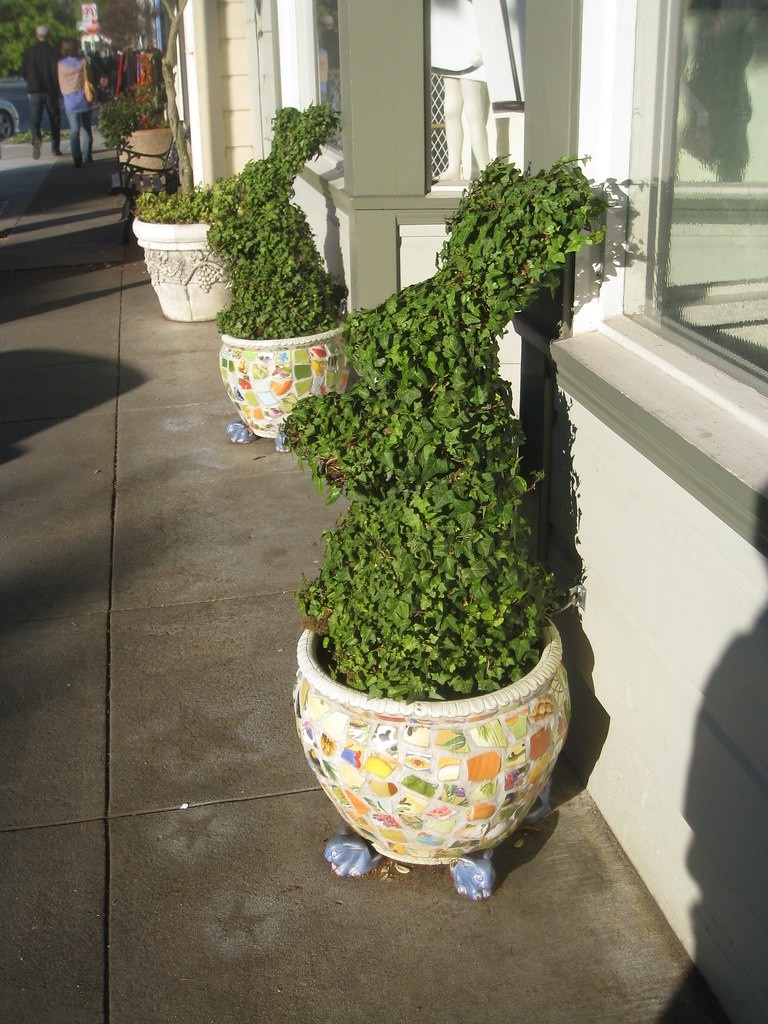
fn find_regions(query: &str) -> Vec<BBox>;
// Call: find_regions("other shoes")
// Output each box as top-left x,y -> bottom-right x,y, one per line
52,150 -> 63,156
83,159 -> 95,164
32,137 -> 41,160
75,156 -> 82,168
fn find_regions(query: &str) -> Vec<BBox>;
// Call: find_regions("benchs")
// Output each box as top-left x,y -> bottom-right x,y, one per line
109,121 -> 189,246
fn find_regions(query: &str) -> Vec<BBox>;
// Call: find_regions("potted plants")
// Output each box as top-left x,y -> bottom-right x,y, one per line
278,152 -> 652,866
204,105 -> 351,439
133,184 -> 232,322
96,80 -> 176,176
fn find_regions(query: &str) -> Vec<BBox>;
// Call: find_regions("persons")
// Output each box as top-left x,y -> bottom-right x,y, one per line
433,77 -> 490,182
57,37 -> 110,168
675,1 -> 757,182
21,23 -> 64,160
318,14 -> 339,69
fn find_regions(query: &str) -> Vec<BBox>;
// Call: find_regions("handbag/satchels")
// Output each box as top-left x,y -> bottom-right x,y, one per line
81,57 -> 95,102
